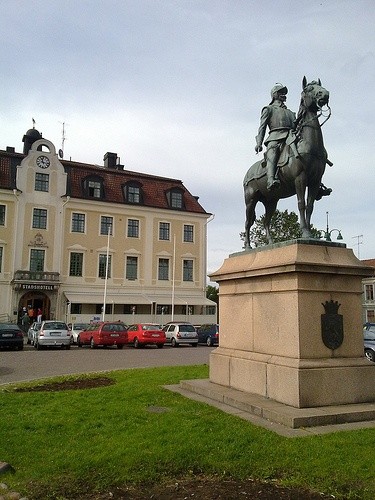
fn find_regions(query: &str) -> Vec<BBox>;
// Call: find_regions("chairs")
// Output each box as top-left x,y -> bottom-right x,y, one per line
45,325 -> 50,328
57,325 -> 62,328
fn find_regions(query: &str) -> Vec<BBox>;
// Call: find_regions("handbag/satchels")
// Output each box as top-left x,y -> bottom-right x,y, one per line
22,320 -> 24,325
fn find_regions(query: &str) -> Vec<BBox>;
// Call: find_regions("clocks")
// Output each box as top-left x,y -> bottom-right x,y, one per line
36,155 -> 51,169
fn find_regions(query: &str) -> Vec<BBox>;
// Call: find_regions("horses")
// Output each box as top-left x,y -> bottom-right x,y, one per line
238,75 -> 332,251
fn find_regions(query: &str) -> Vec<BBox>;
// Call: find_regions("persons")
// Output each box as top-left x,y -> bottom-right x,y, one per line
255,82 -> 332,201
20,305 -> 42,336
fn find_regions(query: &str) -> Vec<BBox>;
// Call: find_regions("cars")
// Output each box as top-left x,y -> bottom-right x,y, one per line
361,322 -> 375,363
77,319 -> 130,349
26,321 -> 42,347
161,321 -> 199,348
197,323 -> 219,346
126,323 -> 166,349
33,320 -> 72,350
67,323 -> 92,345
0,323 -> 24,351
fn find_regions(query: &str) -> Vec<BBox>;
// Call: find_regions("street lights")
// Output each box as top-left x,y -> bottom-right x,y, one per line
314,212 -> 344,242
